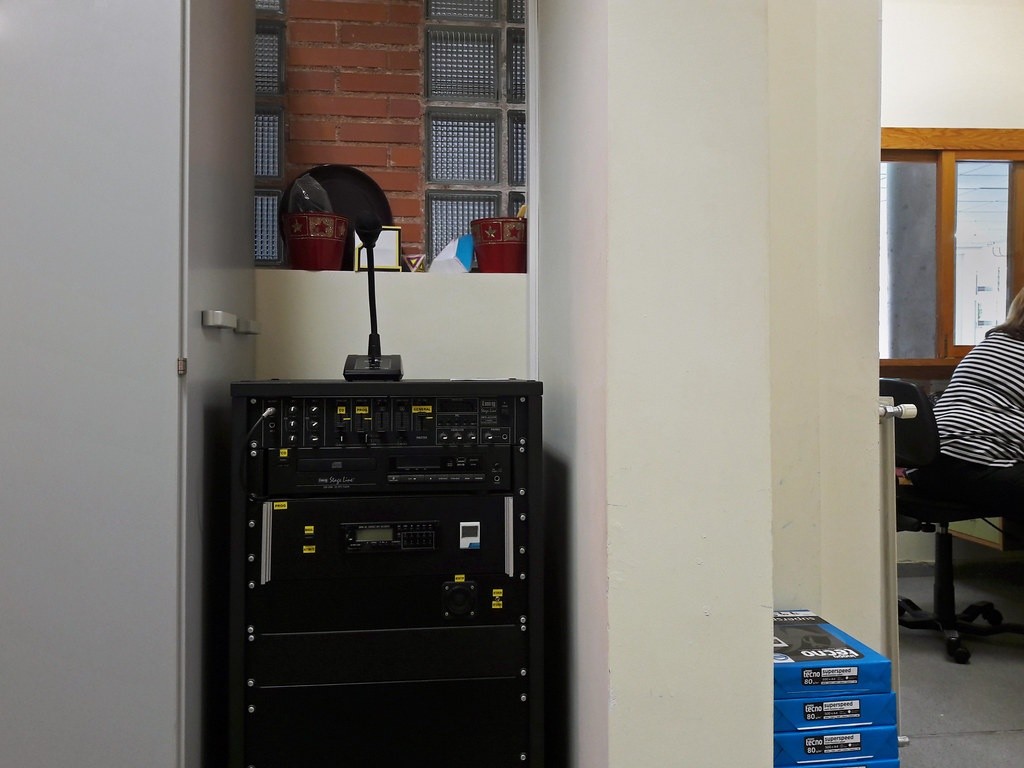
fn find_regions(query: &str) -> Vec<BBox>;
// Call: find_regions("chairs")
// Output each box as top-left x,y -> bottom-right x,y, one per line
879,377 -> 1024,664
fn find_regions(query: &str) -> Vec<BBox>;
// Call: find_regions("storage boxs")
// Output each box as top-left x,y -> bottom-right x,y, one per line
774,609 -> 891,700
773,688 -> 897,733
787,757 -> 900,768
773,723 -> 900,768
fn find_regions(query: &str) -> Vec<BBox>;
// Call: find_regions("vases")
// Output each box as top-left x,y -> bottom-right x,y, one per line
470,217 -> 527,273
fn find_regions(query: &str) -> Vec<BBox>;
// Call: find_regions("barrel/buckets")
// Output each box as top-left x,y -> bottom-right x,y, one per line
284,213 -> 351,270
471,218 -> 528,273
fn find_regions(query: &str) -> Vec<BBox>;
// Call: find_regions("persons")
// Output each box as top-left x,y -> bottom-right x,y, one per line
904,285 -> 1024,553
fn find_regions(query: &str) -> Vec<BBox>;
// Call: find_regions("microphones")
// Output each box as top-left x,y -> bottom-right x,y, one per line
356,210 -> 382,357
